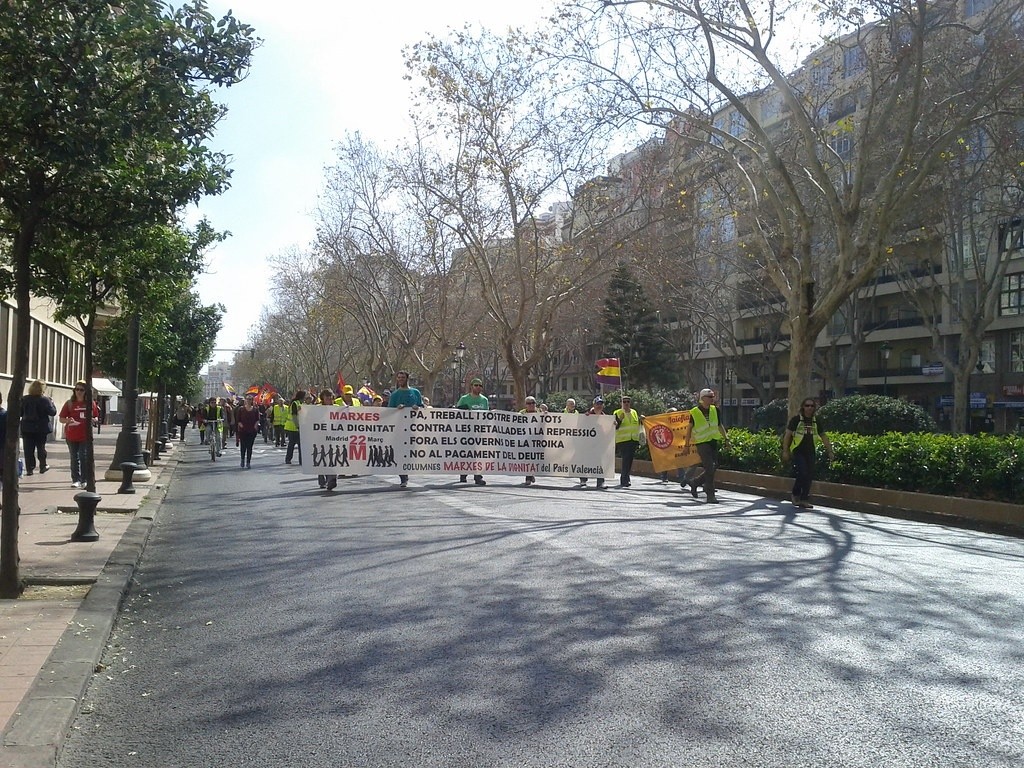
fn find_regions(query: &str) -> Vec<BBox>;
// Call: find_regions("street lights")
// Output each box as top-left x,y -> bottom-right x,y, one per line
539,373 -> 544,399
881,341 -> 892,395
451,342 -> 466,407
715,366 -> 732,423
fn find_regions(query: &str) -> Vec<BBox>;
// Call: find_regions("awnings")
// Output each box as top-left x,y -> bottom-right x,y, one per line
92,378 -> 122,396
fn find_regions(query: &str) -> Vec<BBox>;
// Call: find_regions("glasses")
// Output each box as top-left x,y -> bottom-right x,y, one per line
623,400 -> 630,403
474,384 -> 484,387
74,388 -> 85,392
526,402 -> 536,405
704,395 -> 716,398
804,404 -> 816,407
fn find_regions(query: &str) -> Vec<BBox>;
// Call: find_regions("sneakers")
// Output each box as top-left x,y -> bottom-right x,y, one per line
81,481 -> 88,489
71,480 -> 80,488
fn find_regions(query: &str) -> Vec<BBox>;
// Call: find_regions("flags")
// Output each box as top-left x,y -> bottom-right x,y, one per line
595,358 -> 620,386
246,386 -> 258,396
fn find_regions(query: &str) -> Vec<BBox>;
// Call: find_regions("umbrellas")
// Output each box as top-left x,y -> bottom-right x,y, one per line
138,391 -> 183,401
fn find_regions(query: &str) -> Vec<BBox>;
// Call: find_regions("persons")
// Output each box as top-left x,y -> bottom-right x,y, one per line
0,393 -> 21,512
298,388 -> 349,490
455,378 -> 489,484
580,396 -> 617,489
20,379 -> 57,476
176,385 -> 391,479
59,380 -> 99,488
518,396 -> 549,485
783,397 -> 833,508
613,396 -> 646,486
387,370 -> 424,487
540,404 -> 548,413
560,398 -> 579,414
662,388 -> 730,503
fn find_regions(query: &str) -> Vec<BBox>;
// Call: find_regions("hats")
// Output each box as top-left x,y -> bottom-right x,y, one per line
592,396 -> 604,405
384,391 -> 391,396
471,378 -> 482,385
698,389 -> 714,403
371,394 -> 383,403
342,384 -> 353,395
219,397 -> 226,400
623,396 -> 631,400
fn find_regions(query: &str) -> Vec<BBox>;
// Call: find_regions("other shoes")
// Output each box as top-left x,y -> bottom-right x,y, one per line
681,479 -> 686,488
706,495 -> 720,504
661,479 -> 668,485
460,477 -> 467,483
580,484 -> 587,489
526,476 -> 535,485
285,460 -> 291,464
800,500 -> 814,508
400,479 -> 408,487
689,478 -> 698,498
791,494 -> 801,505
319,482 -> 325,488
475,478 -> 486,484
623,479 -> 631,486
40,463 -> 49,473
241,459 -> 246,467
327,481 -> 337,490
597,483 -> 608,488
245,461 -> 251,469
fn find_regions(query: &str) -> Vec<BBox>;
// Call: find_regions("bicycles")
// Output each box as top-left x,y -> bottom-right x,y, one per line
206,419 -> 222,463
